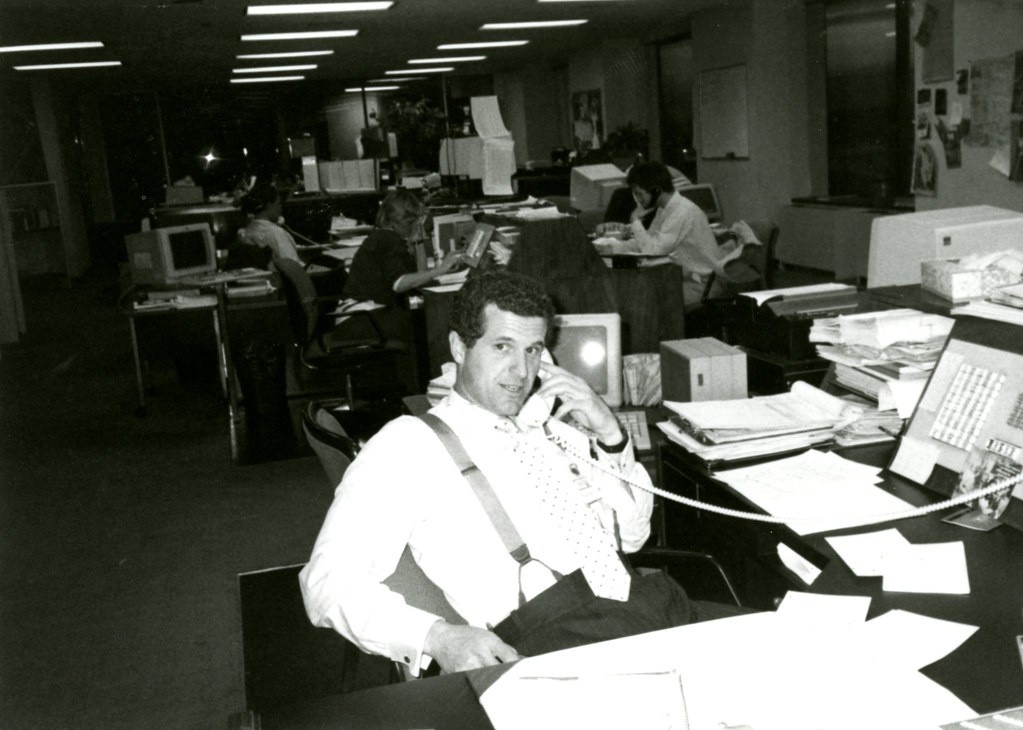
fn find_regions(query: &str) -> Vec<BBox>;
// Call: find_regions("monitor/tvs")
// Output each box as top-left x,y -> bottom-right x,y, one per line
569,163 -> 627,213
126,223 -> 217,286
533,312 -> 623,409
679,183 -> 722,219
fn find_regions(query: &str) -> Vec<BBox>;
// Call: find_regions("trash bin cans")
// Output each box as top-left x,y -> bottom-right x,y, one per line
235,345 -> 290,425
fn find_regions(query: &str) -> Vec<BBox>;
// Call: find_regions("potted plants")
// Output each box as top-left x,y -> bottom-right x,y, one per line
370,95 -> 450,170
603,118 -> 650,170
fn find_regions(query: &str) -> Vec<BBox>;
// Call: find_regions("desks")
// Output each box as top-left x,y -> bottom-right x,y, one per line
402,394 -> 662,546
161,201 -> 242,236
288,190 -> 383,243
229,439 -> 1023,730
416,282 -> 460,376
514,170 -> 568,199
119,266 -> 277,425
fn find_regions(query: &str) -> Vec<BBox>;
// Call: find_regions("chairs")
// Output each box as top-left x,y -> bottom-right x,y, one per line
684,225 -> 783,343
302,400 -> 364,491
275,257 -> 401,410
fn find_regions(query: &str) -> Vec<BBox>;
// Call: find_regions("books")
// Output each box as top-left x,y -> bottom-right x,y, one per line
655,381 -> 851,462
835,363 -> 932,399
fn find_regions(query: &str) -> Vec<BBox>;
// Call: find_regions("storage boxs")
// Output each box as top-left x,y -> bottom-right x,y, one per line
166,186 -> 204,207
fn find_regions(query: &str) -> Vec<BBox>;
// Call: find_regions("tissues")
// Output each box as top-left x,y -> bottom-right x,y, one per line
919,252 -> 986,302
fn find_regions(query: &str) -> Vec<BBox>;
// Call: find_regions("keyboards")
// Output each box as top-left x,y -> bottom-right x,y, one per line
558,412 -> 652,450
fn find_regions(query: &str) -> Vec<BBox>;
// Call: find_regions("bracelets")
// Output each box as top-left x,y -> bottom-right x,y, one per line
596,429 -> 629,454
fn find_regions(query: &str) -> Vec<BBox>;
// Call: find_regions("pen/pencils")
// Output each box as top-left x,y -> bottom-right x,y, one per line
440,251 -> 465,260
879,426 -> 899,439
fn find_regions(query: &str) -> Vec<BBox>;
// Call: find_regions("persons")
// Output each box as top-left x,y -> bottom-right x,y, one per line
597,160 -> 726,306
298,274 -> 761,676
230,184 -> 319,325
330,191 -> 469,396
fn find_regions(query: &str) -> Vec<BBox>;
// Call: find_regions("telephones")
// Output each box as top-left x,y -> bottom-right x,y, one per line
645,185 -> 660,220
247,213 -> 285,224
518,347 -> 556,426
